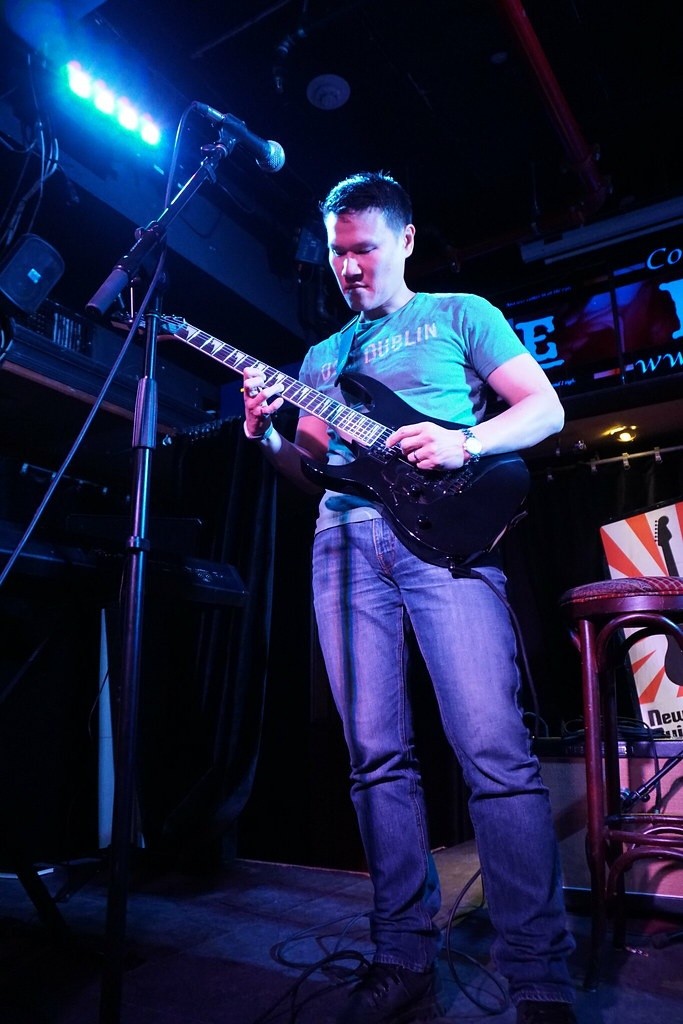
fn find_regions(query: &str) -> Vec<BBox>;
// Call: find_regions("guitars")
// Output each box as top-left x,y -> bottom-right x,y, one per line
110,308 -> 531,568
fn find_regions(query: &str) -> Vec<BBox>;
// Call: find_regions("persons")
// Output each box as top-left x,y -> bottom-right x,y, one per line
244,173 -> 579,1024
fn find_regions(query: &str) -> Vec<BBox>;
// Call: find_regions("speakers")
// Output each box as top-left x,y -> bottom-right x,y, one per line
0,233 -> 66,315
532,736 -> 683,914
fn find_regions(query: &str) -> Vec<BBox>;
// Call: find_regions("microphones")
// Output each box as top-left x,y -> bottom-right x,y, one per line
191,101 -> 285,173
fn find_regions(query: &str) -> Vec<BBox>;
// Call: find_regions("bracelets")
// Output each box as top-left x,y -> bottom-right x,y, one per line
244,420 -> 273,441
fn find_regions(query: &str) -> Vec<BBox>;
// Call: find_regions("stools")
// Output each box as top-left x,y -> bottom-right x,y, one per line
557,577 -> 683,994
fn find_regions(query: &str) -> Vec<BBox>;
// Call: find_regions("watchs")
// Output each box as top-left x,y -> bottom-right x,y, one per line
460,429 -> 483,465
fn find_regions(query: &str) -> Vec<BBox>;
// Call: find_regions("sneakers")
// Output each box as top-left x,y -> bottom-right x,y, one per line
336,961 -> 436,1024
518,1000 -> 576,1024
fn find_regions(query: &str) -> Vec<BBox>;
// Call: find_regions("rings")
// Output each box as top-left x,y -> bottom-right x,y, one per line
414,450 -> 421,463
